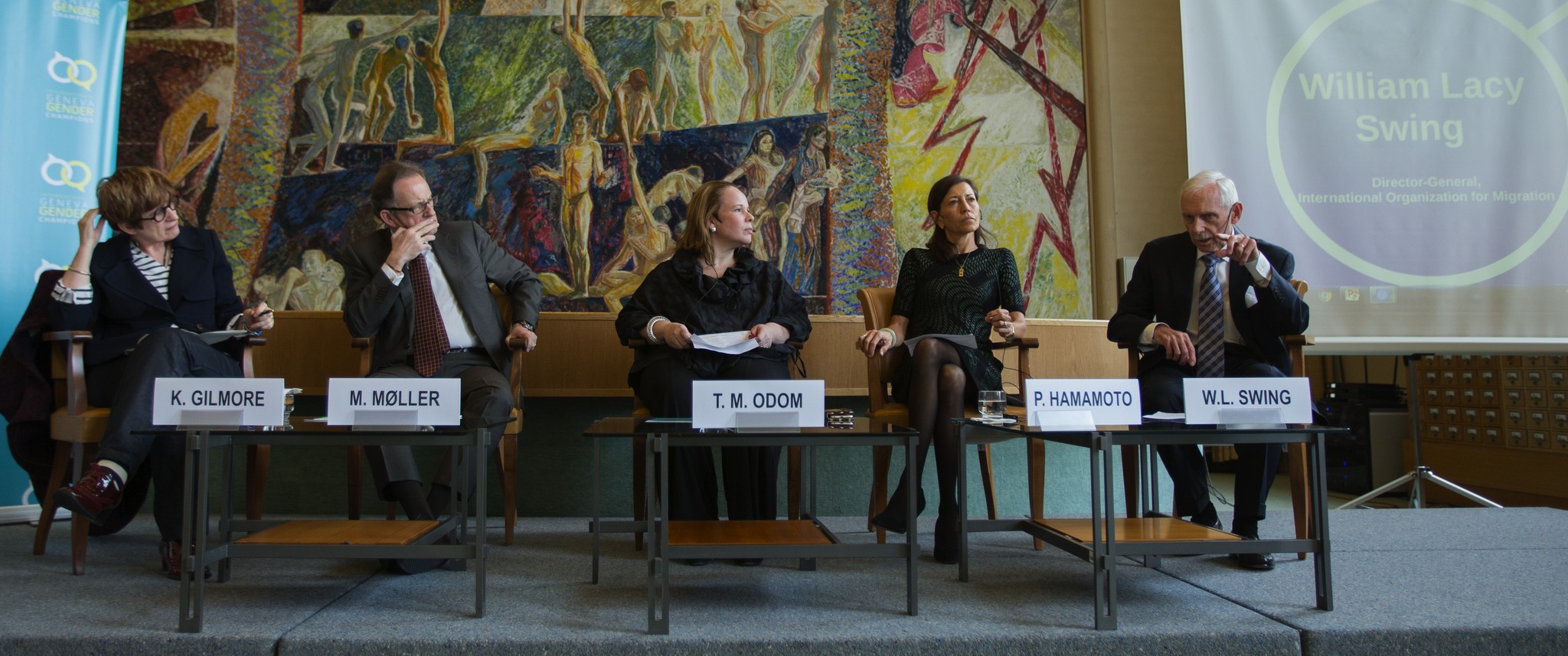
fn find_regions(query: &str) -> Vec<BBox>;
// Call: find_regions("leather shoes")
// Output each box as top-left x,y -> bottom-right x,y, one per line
1229,531 -> 1276,569
60,465 -> 127,528
160,538 -> 212,579
1174,517 -> 1223,556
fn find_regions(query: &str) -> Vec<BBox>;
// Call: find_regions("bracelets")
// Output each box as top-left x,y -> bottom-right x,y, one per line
385,260 -> 402,273
241,316 -> 264,336
878,327 -> 897,350
69,265 -> 92,275
646,315 -> 671,345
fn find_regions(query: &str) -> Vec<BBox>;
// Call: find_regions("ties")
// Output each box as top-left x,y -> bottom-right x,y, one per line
408,254 -> 450,378
1196,253 -> 1226,378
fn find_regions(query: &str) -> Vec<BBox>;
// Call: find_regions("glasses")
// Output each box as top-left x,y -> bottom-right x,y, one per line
140,194 -> 180,222
387,195 -> 439,215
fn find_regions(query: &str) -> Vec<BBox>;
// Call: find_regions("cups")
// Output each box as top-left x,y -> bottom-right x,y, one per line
978,391 -> 1007,420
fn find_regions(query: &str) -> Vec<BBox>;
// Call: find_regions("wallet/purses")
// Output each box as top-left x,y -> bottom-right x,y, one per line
826,409 -> 854,429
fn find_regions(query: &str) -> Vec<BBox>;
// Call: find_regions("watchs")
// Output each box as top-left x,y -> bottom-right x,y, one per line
512,320 -> 535,333
1004,322 -> 1016,340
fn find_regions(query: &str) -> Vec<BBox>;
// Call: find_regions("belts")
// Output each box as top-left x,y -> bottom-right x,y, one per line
450,347 -> 483,354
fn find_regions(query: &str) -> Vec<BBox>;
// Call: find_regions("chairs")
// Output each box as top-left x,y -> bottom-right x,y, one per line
1113,255 -> 1316,563
627,336 -> 801,547
854,287 -> 1046,553
32,273 -> 271,576
350,282 -> 529,549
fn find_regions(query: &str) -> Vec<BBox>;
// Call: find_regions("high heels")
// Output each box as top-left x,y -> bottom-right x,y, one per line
934,507 -> 958,565
872,489 -> 926,534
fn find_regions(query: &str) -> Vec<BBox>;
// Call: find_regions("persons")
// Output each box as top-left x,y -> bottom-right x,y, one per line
858,174 -> 1027,564
342,158 -> 546,575
1106,169 -> 1310,570
614,181 -> 814,567
47,166 -> 275,580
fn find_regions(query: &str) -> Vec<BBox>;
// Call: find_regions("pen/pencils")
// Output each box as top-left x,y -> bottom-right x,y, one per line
1003,414 -> 1019,419
253,309 -> 274,317
749,331 -> 756,337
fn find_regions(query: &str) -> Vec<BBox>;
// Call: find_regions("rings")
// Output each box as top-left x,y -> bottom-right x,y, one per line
535,344 -> 536,346
861,333 -> 866,338
762,345 -> 766,348
1005,321 -> 1007,325
1238,243 -> 1244,248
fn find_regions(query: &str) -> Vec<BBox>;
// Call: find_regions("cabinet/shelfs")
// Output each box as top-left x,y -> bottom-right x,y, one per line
1405,354 -> 1568,499
950,411 -> 1351,632
131,408 -> 517,637
582,415 -> 922,634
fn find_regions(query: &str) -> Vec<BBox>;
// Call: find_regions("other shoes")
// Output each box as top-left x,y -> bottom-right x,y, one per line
687,556 -> 709,565
395,554 -> 437,575
735,556 -> 763,565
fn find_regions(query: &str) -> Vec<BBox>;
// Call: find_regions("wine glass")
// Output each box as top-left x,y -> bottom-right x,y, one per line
284,390 -> 295,424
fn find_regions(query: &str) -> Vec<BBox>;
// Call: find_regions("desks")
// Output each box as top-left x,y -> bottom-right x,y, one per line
240,308 -> 1125,545
